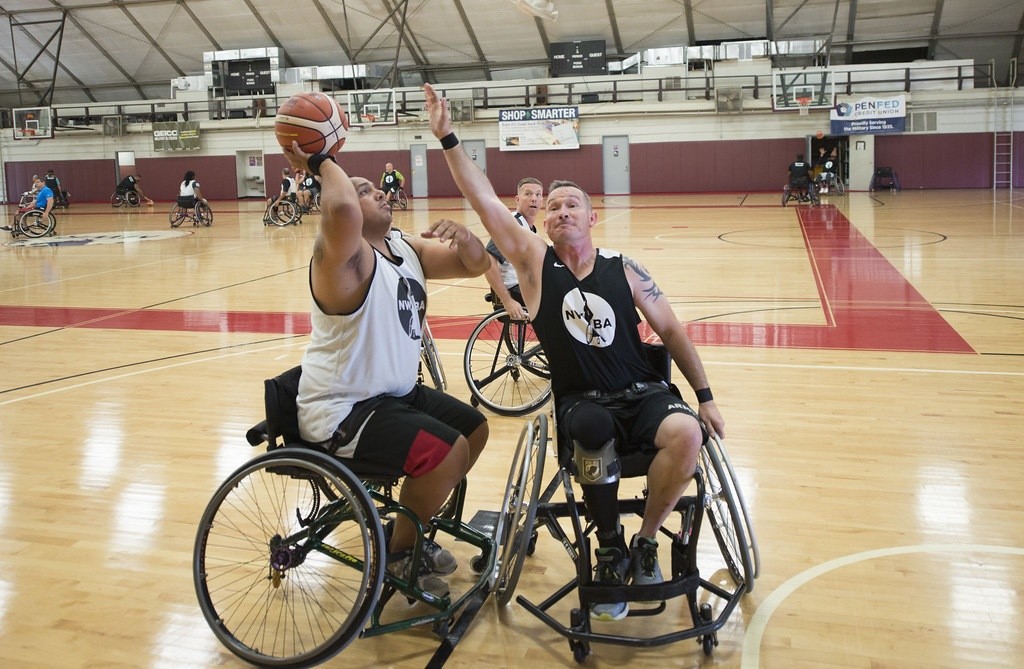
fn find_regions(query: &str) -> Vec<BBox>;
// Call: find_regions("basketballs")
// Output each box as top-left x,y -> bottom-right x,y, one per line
816,130 -> 824,139
147,200 -> 154,207
274,90 -> 349,156
292,165 -> 301,173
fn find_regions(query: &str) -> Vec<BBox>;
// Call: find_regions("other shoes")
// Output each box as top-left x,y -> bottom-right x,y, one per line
18,226 -> 29,231
0,226 -> 12,231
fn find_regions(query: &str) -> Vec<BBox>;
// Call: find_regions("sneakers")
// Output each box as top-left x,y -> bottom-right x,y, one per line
590,537 -> 632,622
629,536 -> 664,604
387,522 -> 457,573
387,551 -> 450,599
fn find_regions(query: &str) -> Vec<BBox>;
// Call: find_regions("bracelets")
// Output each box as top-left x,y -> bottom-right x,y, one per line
296,171 -> 300,173
307,154 -> 338,177
399,187 -> 403,190
440,132 -> 459,150
695,387 -> 713,404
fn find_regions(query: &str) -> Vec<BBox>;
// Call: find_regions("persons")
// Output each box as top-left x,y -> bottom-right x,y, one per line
424,84 -> 726,620
485,178 -> 544,318
380,163 -> 405,200
118,175 -> 152,203
788,154 -> 814,202
265,167 -> 296,220
294,168 -> 321,213
0,177 -> 54,231
45,170 -> 70,209
19,175 -> 38,206
180,171 -> 208,216
282,141 -> 492,600
819,147 -> 837,193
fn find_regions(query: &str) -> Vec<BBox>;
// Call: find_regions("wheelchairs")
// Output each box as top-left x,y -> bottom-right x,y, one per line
814,169 -> 845,196
302,192 -> 321,215
389,187 -> 408,211
263,192 -> 303,227
869,166 -> 900,192
463,285 -> 552,418
52,188 -> 69,209
11,204 -> 57,239
493,341 -> 759,665
169,195 -> 213,228
782,176 -> 821,207
417,317 -> 448,394
111,185 -> 141,208
193,365 -> 498,669
